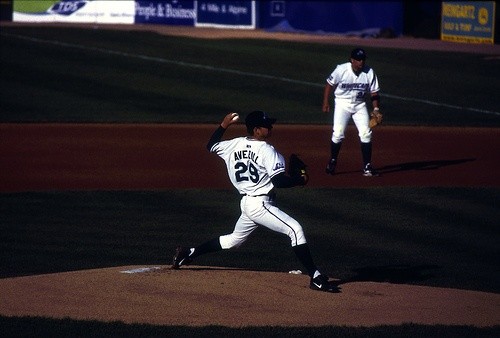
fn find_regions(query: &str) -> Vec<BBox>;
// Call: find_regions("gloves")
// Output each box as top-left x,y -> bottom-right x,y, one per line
290,153 -> 308,187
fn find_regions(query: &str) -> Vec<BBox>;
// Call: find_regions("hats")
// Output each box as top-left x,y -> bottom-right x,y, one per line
246,110 -> 278,127
350,48 -> 366,61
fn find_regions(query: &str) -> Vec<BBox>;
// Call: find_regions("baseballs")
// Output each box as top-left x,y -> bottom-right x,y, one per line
231,113 -> 239,121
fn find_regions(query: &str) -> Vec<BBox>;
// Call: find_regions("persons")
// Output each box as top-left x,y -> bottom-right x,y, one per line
172,111 -> 341,294
321,48 -> 383,178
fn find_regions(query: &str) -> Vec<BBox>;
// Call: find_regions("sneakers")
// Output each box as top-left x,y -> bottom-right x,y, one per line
327,157 -> 337,176
360,160 -> 379,177
310,277 -> 342,292
171,243 -> 193,268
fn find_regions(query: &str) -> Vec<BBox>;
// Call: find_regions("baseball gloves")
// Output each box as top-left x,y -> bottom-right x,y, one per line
368,113 -> 382,133
288,154 -> 310,177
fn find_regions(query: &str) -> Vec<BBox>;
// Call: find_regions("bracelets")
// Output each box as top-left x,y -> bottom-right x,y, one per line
373,107 -> 379,112
